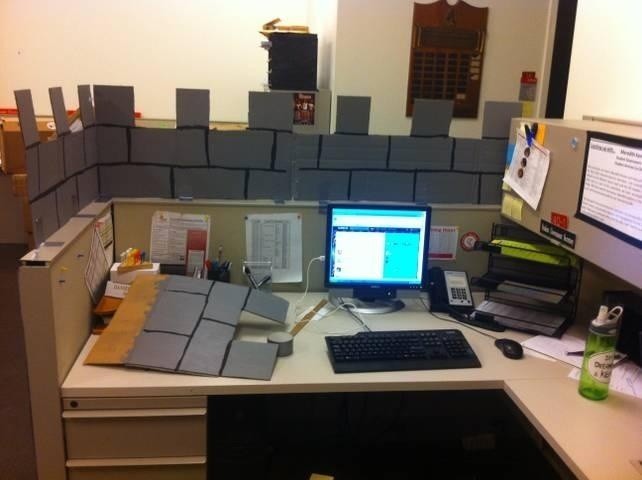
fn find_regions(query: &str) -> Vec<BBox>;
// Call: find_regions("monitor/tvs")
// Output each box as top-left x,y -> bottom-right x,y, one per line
323,204 -> 432,314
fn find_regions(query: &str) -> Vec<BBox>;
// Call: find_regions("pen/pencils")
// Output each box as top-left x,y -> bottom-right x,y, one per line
245,268 -> 271,290
567,352 -> 585,355
205,246 -> 232,272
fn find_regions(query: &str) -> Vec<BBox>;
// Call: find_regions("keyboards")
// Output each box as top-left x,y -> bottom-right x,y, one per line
325,329 -> 482,373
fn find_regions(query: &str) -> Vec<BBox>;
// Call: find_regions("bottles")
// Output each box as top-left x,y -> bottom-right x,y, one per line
576,304 -> 625,402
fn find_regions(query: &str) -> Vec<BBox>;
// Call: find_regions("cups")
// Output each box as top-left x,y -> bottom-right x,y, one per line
207,269 -> 231,283
243,260 -> 272,291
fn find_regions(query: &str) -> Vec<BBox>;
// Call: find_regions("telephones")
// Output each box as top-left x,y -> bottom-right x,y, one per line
428,266 -> 476,313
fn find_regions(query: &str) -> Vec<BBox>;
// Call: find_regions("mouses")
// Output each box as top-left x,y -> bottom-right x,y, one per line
495,339 -> 523,359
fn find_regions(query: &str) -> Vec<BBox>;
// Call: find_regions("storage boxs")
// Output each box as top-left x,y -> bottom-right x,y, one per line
1,114 -> 77,251
